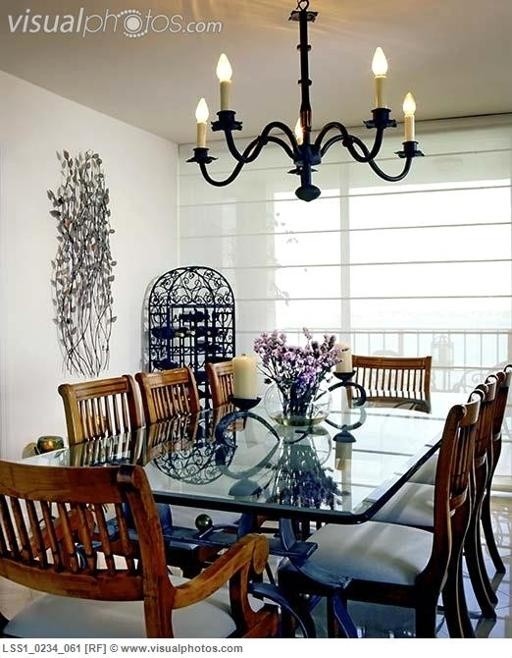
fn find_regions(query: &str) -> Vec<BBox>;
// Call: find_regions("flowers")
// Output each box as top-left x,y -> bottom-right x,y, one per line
251,328 -> 349,416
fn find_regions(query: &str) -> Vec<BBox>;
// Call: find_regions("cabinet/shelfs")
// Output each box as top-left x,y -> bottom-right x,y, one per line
148,266 -> 235,409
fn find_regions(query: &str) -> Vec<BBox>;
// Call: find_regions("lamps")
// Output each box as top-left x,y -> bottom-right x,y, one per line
187,0 -> 424,200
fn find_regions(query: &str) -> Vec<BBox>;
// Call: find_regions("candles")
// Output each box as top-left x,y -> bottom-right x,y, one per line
232,354 -> 255,400
337,346 -> 352,371
